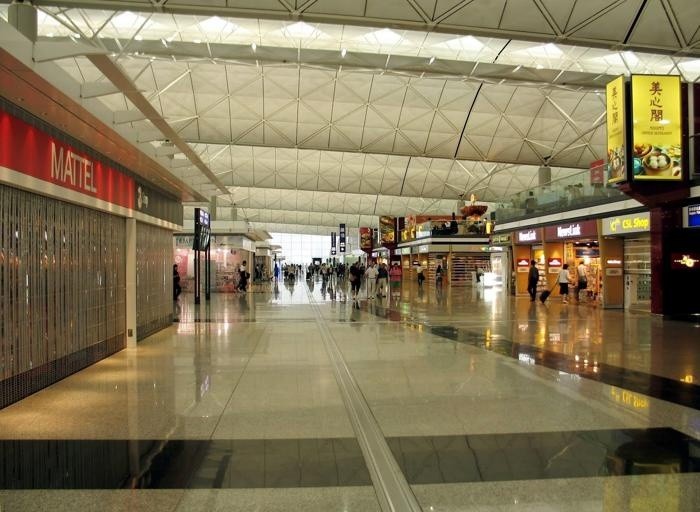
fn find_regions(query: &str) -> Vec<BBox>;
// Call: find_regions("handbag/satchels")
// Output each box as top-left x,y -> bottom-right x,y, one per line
578,280 -> 588,290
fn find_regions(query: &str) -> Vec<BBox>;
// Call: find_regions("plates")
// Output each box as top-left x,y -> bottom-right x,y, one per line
634,144 -> 655,159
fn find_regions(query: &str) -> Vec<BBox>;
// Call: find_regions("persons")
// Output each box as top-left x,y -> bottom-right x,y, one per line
555,264 -> 574,304
436,264 -> 443,276
253,260 -> 402,303
478,221 -> 483,230
234,260 -> 247,292
527,260 -> 539,302
477,218 -> 487,235
525,191 -> 537,214
574,261 -> 588,301
173,264 -> 182,300
417,264 -> 423,286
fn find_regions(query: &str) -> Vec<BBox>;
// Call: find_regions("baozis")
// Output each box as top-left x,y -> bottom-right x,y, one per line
649,155 -> 667,169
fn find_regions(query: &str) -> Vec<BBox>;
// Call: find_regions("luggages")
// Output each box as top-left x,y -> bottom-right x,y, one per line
538,282 -> 558,304
